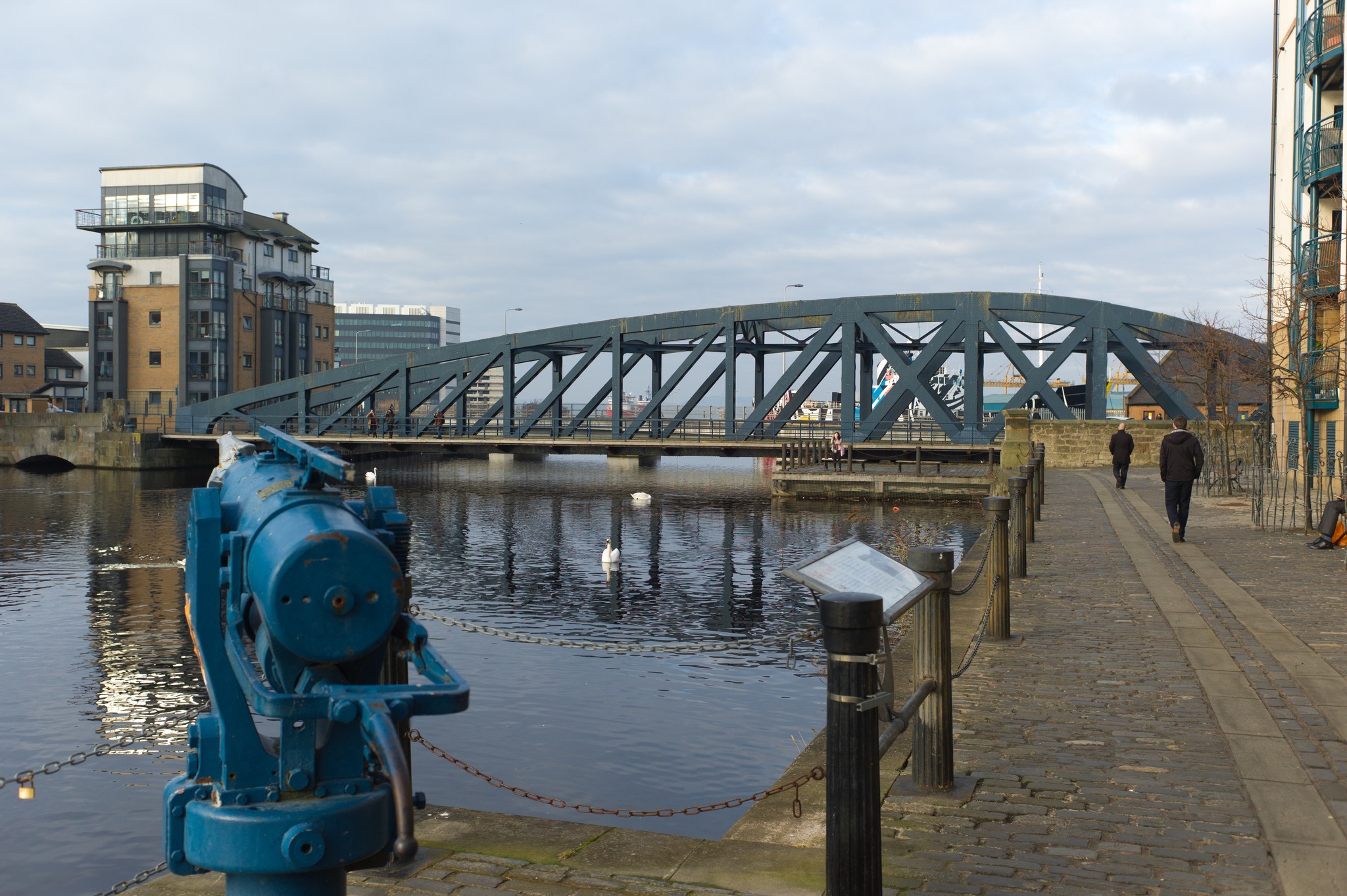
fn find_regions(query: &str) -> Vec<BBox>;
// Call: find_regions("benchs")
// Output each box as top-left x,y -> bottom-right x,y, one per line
821,458 -> 867,472
895,460 -> 942,474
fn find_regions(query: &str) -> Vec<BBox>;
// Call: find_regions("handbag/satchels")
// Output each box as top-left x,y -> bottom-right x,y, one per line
392,419 -> 395,424
1318,519 -> 1347,546
840,438 -> 845,457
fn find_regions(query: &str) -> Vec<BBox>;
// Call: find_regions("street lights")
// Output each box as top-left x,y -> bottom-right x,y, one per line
355,330 -> 372,363
782,284 -> 804,407
204,335 -> 219,398
504,308 -> 523,335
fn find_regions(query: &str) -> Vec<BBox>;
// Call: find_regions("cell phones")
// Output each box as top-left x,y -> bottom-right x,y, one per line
1334,495 -> 1345,500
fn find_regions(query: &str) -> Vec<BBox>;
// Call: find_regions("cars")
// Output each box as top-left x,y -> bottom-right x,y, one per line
47,402 -> 74,413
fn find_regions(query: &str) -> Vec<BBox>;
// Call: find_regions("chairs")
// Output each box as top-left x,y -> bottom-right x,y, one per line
1333,511 -> 1347,547
1209,457 -> 1245,491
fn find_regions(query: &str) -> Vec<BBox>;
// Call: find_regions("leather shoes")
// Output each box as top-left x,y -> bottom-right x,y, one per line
1305,538 -> 1321,546
1308,540 -> 1334,550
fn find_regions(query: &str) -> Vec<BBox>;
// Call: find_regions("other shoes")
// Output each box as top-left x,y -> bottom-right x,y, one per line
373,436 -> 375,438
1120,486 -> 1125,489
387,435 -> 393,439
1179,538 -> 1184,542
436,437 -> 440,439
376,437 -> 377,438
837,468 -> 841,472
1116,477 -> 1121,488
438,437 -> 442,439
833,468 -> 836,472
1172,522 -> 1180,543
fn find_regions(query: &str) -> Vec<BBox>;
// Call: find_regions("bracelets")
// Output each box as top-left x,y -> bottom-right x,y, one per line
832,438 -> 834,439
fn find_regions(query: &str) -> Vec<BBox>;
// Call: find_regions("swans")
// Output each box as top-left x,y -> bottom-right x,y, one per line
364,467 -> 377,477
601,539 -> 620,562
630,492 -> 652,500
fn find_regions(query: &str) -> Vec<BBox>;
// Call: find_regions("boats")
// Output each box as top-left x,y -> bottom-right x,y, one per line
605,384 -> 652,417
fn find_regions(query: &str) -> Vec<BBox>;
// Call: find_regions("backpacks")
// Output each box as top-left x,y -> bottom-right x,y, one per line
441,415 -> 445,424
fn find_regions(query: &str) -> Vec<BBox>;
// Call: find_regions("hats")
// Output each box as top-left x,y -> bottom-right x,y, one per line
436,409 -> 440,412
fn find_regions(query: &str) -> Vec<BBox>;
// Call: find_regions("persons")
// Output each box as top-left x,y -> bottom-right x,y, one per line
1143,412 -> 1162,420
1304,491 -> 1347,549
366,409 -> 443,439
1027,409 -> 1041,420
1108,423 -> 1135,490
1159,415 -> 1204,543
46,403 -> 58,413
830,432 -> 842,472
